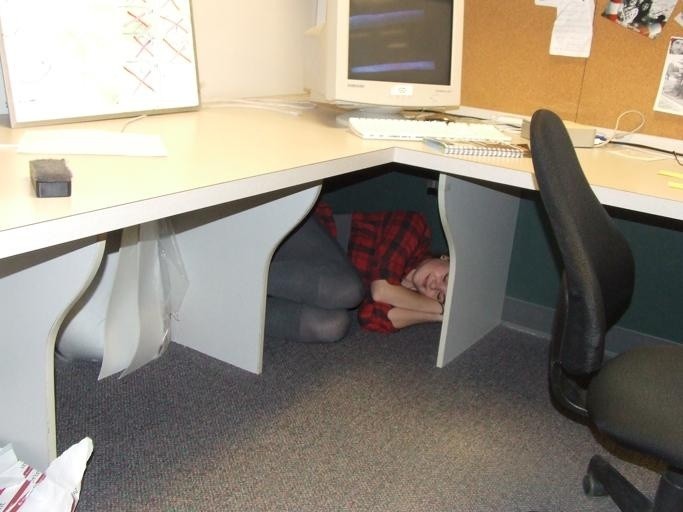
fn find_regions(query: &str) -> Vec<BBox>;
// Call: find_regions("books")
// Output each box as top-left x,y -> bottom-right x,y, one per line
423,135 -> 525,159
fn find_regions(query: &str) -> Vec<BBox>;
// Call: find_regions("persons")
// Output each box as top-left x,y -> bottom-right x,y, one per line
265,203 -> 450,346
646,14 -> 666,40
669,39 -> 683,55
635,0 -> 653,25
620,0 -> 641,28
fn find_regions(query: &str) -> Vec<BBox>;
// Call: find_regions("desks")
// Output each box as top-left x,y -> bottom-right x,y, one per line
0,95 -> 682,475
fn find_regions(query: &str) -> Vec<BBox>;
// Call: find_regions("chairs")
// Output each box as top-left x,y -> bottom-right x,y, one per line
532,109 -> 683,511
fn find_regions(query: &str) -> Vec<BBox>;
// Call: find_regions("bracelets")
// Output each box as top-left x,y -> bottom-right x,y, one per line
438,301 -> 445,316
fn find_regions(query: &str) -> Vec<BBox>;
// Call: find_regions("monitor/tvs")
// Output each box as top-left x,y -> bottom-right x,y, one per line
310,0 -> 464,126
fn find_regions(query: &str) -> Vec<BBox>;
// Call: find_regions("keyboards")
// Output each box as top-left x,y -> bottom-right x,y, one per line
348,117 -> 512,145
